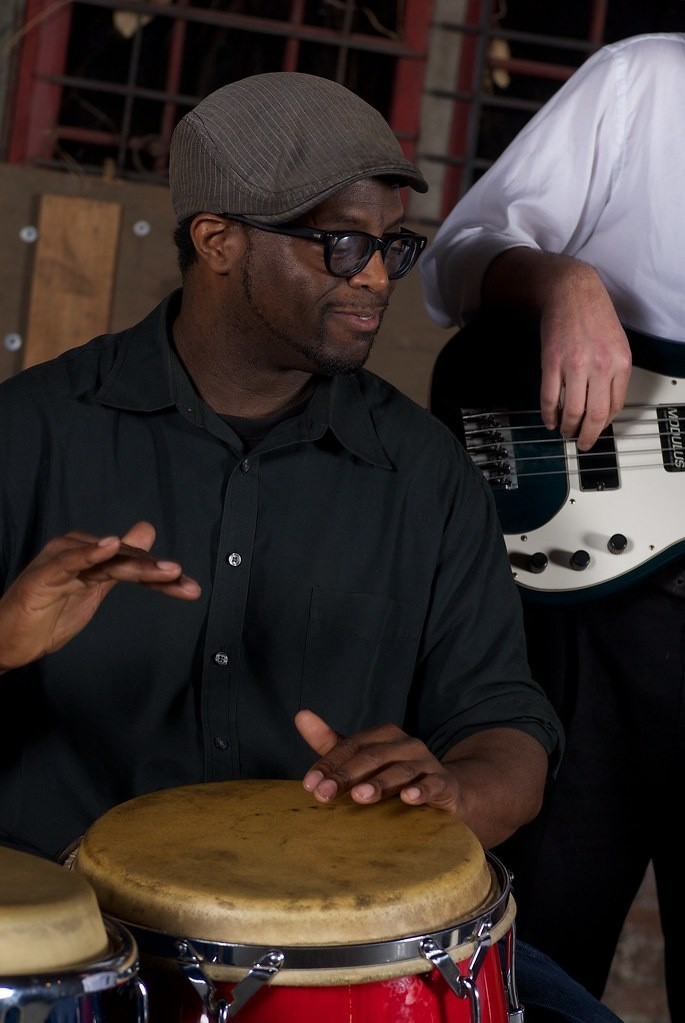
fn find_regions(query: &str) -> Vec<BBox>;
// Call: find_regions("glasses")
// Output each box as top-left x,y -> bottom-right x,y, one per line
217,212 -> 428,280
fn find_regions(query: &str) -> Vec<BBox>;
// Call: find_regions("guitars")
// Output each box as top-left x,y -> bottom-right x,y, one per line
427,315 -> 684,611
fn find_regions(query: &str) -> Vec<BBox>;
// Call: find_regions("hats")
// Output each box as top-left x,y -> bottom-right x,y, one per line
167,73 -> 428,227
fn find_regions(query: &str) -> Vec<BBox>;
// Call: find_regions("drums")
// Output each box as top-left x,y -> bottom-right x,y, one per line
1,840 -> 144,1022
53,777 -> 518,1023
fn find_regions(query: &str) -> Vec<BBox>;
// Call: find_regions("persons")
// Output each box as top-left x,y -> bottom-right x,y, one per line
0,72 -> 618,1023
417,30 -> 685,1023
